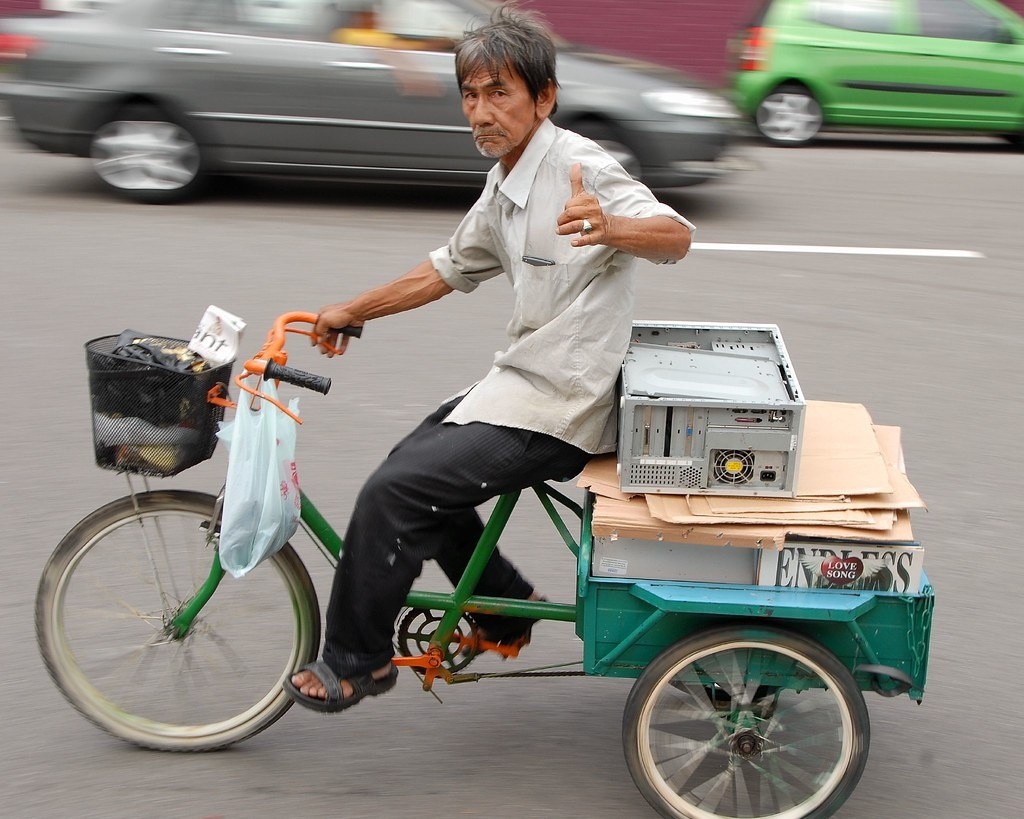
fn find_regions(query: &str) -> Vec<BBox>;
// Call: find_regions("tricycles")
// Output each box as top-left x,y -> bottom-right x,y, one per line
32,310 -> 936,817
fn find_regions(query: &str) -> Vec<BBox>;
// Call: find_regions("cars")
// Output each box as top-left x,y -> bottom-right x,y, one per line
727,1 -> 1023,151
1,0 -> 743,204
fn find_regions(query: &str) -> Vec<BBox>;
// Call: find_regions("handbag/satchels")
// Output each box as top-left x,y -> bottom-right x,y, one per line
216,365 -> 301,577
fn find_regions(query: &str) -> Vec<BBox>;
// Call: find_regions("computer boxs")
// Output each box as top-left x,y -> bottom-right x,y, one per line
617,320 -> 805,498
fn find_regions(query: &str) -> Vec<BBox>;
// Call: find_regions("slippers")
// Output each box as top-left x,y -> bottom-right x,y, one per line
462,597 -> 549,658
281,661 -> 397,713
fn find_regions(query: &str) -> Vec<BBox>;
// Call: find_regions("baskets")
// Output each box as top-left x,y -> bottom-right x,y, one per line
83,334 -> 236,478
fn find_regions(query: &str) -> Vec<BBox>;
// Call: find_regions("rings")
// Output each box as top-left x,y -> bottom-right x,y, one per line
582,219 -> 592,233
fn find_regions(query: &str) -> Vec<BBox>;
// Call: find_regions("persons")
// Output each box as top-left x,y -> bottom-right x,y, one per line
282,0 -> 696,711
